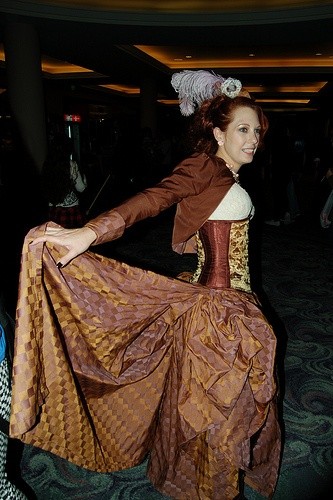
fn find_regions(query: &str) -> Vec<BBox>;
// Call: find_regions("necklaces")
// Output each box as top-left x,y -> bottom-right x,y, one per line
226,163 -> 240,182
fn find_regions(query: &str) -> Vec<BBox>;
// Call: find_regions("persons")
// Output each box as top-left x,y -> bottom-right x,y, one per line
10,69 -> 282,500
